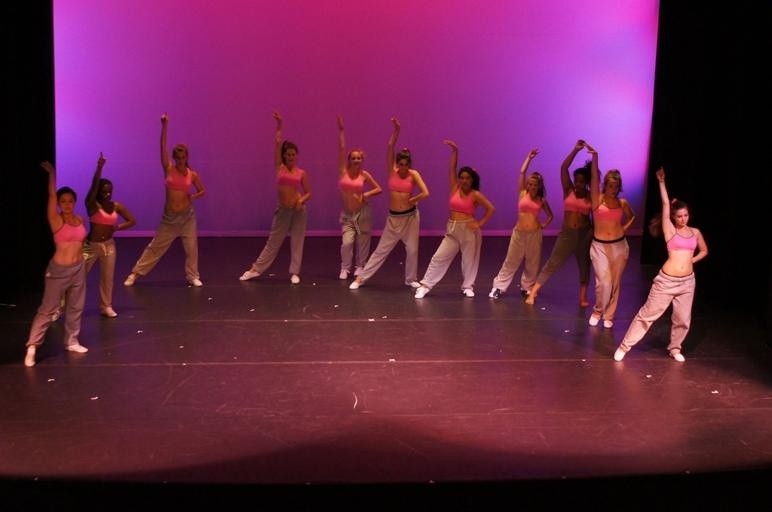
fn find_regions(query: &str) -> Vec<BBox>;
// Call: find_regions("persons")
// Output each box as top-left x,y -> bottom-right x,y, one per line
488,147 -> 554,299
586,144 -> 636,328
334,111 -> 383,280
22,160 -> 89,367
49,150 -> 137,322
348,114 -> 430,290
413,139 -> 496,300
614,168 -> 708,361
239,109 -> 313,285
122,110 -> 206,288
525,139 -> 592,307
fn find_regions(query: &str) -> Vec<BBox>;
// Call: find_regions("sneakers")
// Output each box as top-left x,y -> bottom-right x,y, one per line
239,271 -> 260,281
102,306 -> 118,318
603,320 -> 614,328
588,315 -> 599,326
189,279 -> 204,287
24,347 -> 36,368
487,288 -> 503,300
669,353 -> 685,362
614,348 -> 625,361
123,274 -> 137,287
64,343 -> 89,353
404,279 -> 432,299
461,286 -> 475,299
290,274 -> 300,284
338,266 -> 370,291
520,288 -> 538,297
